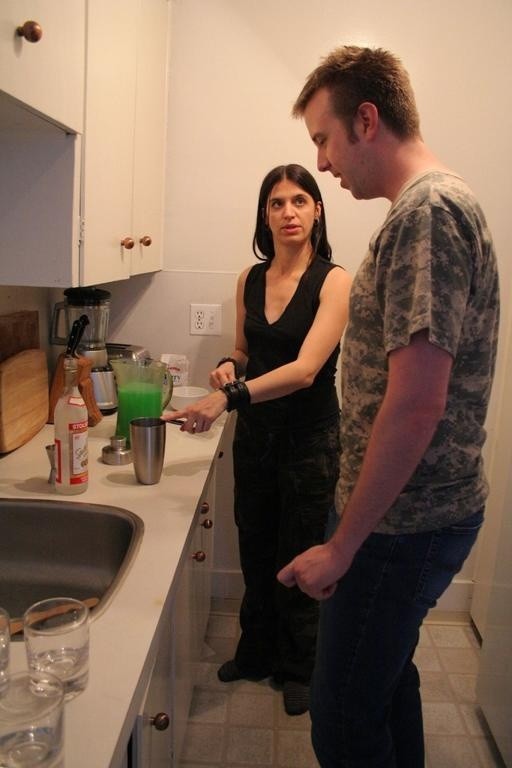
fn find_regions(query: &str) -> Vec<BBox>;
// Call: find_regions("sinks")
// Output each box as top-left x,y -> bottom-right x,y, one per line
0,494 -> 145,643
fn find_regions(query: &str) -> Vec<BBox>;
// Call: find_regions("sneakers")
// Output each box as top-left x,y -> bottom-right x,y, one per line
285,680 -> 310,715
217,659 -> 242,682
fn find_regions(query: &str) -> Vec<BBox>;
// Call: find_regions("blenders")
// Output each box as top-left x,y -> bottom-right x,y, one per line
52,288 -> 119,414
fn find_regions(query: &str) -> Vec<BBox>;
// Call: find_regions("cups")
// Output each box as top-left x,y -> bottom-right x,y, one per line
2,606 -> 17,674
1,673 -> 67,765
20,595 -> 88,704
130,419 -> 168,486
109,356 -> 175,445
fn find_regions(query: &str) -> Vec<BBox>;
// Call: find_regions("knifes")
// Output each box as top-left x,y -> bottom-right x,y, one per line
49,315 -> 103,359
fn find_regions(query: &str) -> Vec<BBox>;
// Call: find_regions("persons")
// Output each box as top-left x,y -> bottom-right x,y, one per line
278,42 -> 501,766
159,166 -> 352,716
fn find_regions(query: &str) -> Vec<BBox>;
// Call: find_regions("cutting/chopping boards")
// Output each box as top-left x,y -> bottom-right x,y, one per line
0,308 -> 40,365
0,346 -> 53,452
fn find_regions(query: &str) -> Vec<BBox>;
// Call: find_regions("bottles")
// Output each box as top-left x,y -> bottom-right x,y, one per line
53,359 -> 91,494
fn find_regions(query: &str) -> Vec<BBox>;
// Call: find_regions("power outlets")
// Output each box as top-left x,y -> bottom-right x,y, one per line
190,305 -> 222,336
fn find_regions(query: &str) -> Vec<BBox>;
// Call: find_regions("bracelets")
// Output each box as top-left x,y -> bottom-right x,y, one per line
215,358 -> 243,380
219,379 -> 252,413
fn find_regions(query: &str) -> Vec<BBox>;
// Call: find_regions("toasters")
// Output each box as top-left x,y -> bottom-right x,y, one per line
106,338 -> 153,369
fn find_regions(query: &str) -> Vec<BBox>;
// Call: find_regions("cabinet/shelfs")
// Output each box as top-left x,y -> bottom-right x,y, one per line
117,454 -> 219,768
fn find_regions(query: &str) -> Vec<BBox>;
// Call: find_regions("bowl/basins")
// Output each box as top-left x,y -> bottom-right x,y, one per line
172,385 -> 211,411
